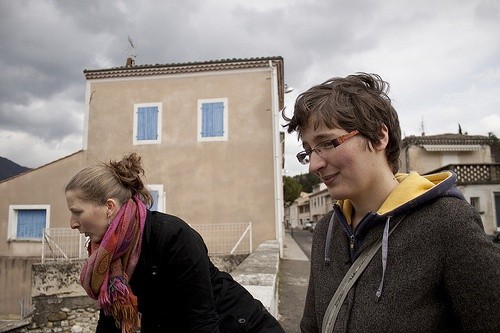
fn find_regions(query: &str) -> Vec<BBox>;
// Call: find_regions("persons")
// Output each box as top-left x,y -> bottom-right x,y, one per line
64,154 -> 284,333
284,73 -> 500,333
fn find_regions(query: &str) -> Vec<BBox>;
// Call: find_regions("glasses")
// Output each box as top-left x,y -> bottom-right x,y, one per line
294,130 -> 361,165
